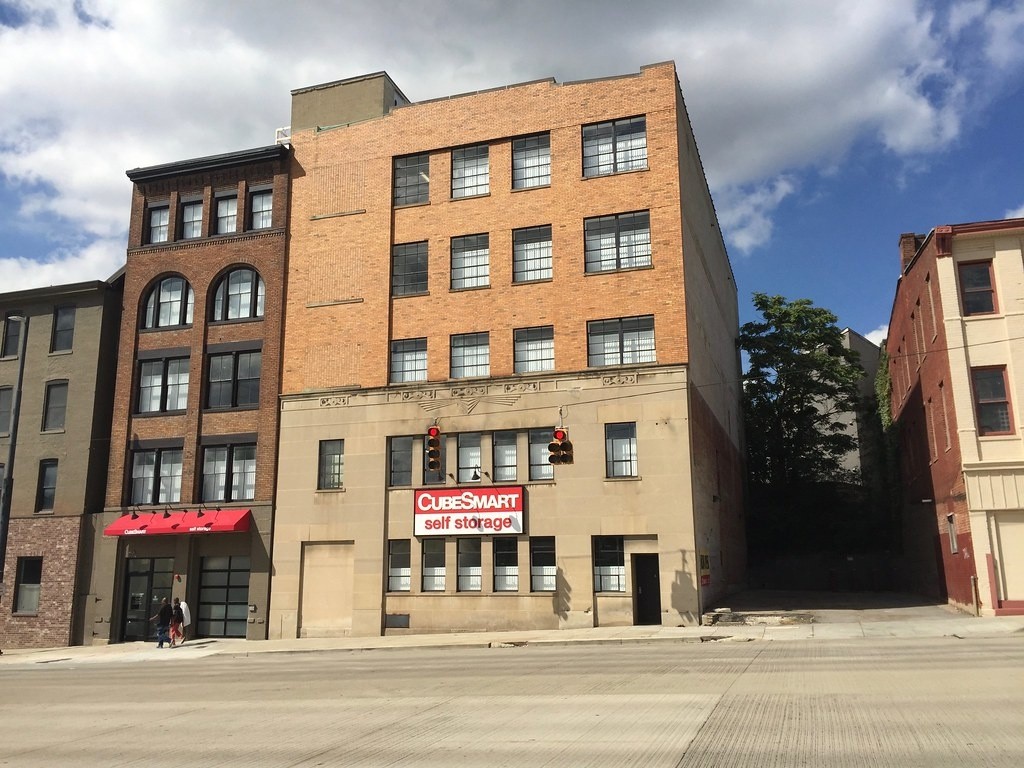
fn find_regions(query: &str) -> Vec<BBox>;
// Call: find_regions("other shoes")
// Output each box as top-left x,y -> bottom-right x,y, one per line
180,636 -> 186,644
169,640 -> 175,648
157,643 -> 163,648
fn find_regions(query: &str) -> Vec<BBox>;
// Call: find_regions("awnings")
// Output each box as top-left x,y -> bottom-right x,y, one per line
103,509 -> 249,536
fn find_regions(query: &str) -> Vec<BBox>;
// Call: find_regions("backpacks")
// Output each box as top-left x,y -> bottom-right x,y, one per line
174,608 -> 183,623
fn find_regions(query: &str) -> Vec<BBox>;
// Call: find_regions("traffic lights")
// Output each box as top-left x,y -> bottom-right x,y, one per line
428,426 -> 442,472
548,427 -> 574,464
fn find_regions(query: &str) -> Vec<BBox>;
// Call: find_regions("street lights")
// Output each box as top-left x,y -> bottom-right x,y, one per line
0,315 -> 32,588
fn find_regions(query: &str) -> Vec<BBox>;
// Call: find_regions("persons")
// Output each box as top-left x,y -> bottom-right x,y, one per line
169,597 -> 186,645
150,598 -> 174,648
